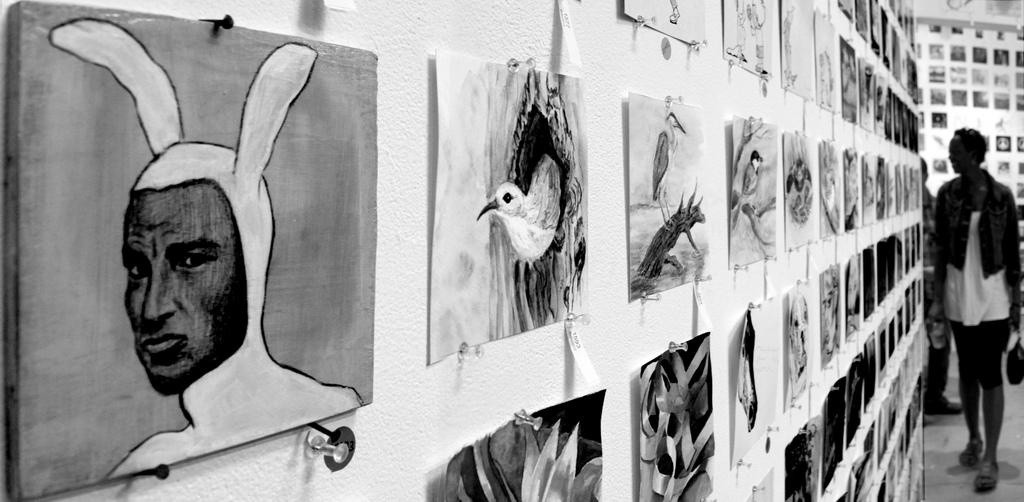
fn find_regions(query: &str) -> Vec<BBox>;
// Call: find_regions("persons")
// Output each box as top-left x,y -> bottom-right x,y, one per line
933,125 -> 1023,499
48,11 -> 365,480
919,155 -> 962,419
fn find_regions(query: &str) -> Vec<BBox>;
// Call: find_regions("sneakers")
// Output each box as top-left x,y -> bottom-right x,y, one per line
973,459 -> 999,492
922,398 -> 964,416
958,439 -> 983,466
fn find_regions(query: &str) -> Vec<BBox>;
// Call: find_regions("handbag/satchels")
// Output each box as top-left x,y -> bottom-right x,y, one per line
1006,337 -> 1024,386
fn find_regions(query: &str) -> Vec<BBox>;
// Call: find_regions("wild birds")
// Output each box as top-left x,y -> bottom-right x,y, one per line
476,153 -> 562,274
651,110 -> 688,234
730,149 -> 764,231
